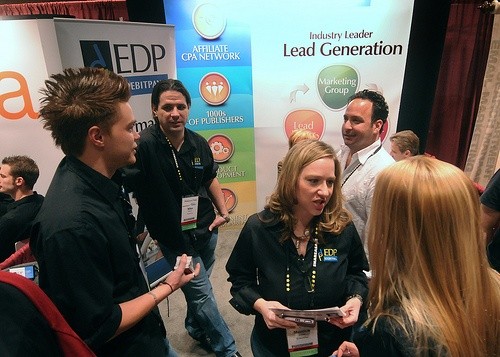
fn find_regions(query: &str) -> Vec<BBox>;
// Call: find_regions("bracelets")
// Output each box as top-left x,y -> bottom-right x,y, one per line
161,280 -> 174,293
219,213 -> 231,225
346,294 -> 364,308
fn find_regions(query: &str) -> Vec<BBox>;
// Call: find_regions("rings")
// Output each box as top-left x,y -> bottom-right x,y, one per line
339,321 -> 343,324
192,272 -> 197,278
347,350 -> 351,355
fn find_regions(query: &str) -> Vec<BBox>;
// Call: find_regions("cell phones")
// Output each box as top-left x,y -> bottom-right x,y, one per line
284,317 -> 315,327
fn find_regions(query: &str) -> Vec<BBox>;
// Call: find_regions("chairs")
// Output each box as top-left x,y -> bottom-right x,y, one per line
0,239 -> 36,268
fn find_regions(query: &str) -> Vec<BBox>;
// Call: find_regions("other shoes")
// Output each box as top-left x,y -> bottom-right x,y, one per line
232,352 -> 242,357
185,317 -> 214,353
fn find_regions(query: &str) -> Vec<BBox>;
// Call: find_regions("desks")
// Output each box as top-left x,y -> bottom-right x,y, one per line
2,260 -> 40,288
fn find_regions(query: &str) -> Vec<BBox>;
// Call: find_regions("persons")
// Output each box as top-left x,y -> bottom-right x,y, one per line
121,79 -> 242,357
225,128 -> 371,357
0,155 -> 97,357
336,90 -> 397,295
328,155 -> 500,357
479,168 -> 500,274
29,67 -> 201,357
389,131 -> 420,162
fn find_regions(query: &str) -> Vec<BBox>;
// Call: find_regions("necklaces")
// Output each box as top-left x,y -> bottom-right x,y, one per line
177,140 -> 185,152
338,144 -> 383,188
291,227 -> 310,248
